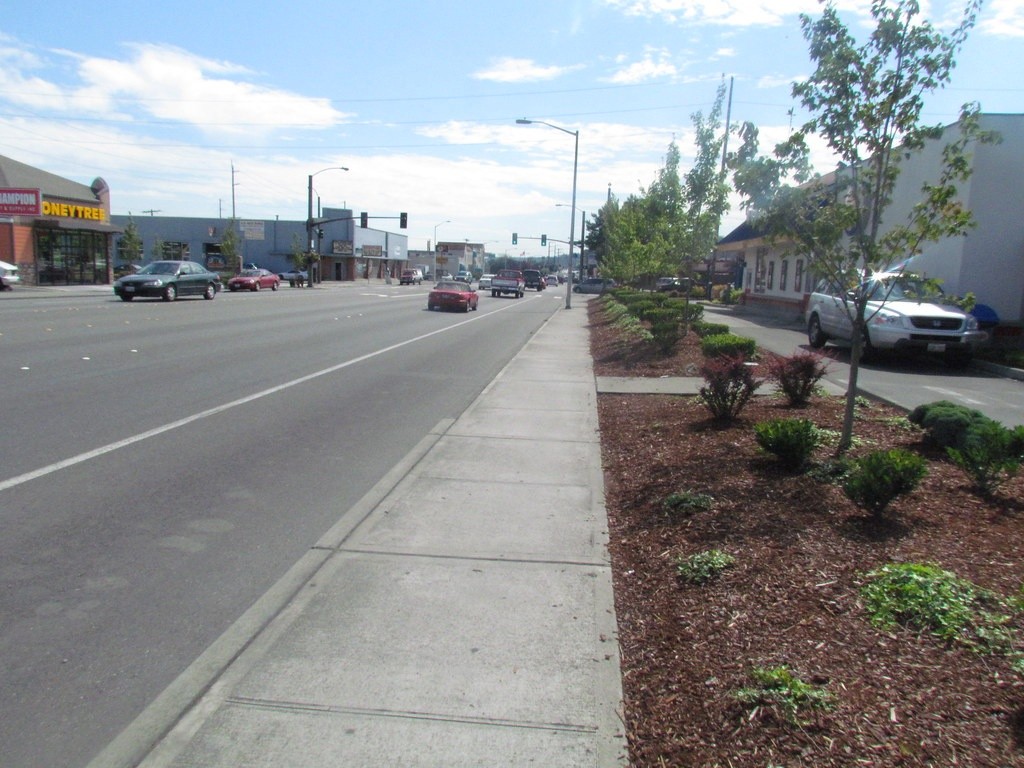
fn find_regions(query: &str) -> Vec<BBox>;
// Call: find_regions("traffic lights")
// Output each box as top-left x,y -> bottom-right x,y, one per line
541,234 -> 547,246
512,233 -> 517,244
319,228 -> 324,239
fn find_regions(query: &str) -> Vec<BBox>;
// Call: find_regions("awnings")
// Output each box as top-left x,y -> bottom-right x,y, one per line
715,196 -> 827,251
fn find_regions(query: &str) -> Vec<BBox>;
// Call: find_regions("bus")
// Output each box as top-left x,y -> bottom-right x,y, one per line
166,251 -> 243,273
572,271 -> 579,280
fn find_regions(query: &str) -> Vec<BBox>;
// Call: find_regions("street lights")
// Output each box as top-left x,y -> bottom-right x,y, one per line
433,219 -> 451,281
307,166 -> 349,288
482,240 -> 499,274
505,247 -> 519,270
517,118 -> 579,308
307,185 -> 322,283
556,202 -> 586,280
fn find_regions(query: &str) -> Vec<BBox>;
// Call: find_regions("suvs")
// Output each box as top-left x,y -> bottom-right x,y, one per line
453,271 -> 473,284
523,269 -> 542,291
803,266 -> 989,363
399,268 -> 423,285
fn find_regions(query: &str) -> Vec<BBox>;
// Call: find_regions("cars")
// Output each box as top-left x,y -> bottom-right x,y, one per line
83,258 -> 108,270
558,274 -> 568,283
541,277 -> 546,289
428,281 -> 479,313
113,261 -> 222,302
655,277 -> 708,294
113,263 -> 150,280
278,269 -> 309,281
441,272 -> 454,280
424,271 -> 434,280
575,277 -> 623,295
226,269 -> 281,292
545,275 -> 559,287
245,263 -> 262,269
478,273 -> 497,290
0,260 -> 18,292
39,261 -> 94,282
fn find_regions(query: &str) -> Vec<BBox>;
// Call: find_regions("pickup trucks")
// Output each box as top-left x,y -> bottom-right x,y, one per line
491,269 -> 525,298
